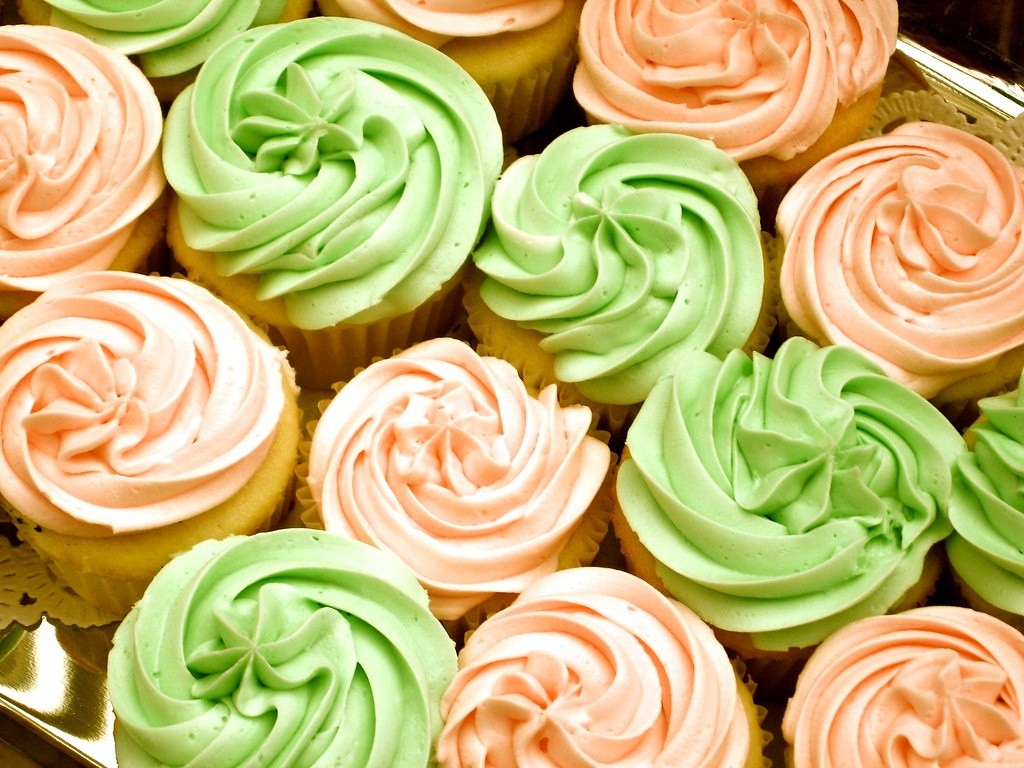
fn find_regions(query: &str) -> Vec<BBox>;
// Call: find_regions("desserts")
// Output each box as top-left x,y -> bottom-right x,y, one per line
0,0 -> 1024,768
